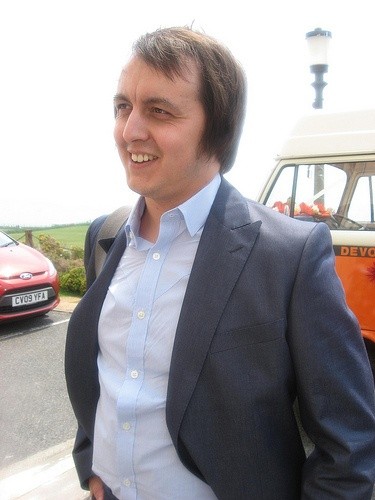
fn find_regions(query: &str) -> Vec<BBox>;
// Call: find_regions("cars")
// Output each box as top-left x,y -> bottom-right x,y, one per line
1,231 -> 63,319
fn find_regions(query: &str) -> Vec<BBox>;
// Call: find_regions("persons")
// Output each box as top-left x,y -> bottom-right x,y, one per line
64,27 -> 374,500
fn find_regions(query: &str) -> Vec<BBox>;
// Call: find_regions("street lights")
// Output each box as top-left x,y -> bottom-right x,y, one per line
304,26 -> 334,205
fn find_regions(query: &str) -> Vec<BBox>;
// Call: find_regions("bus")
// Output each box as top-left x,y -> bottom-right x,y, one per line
259,108 -> 375,376
